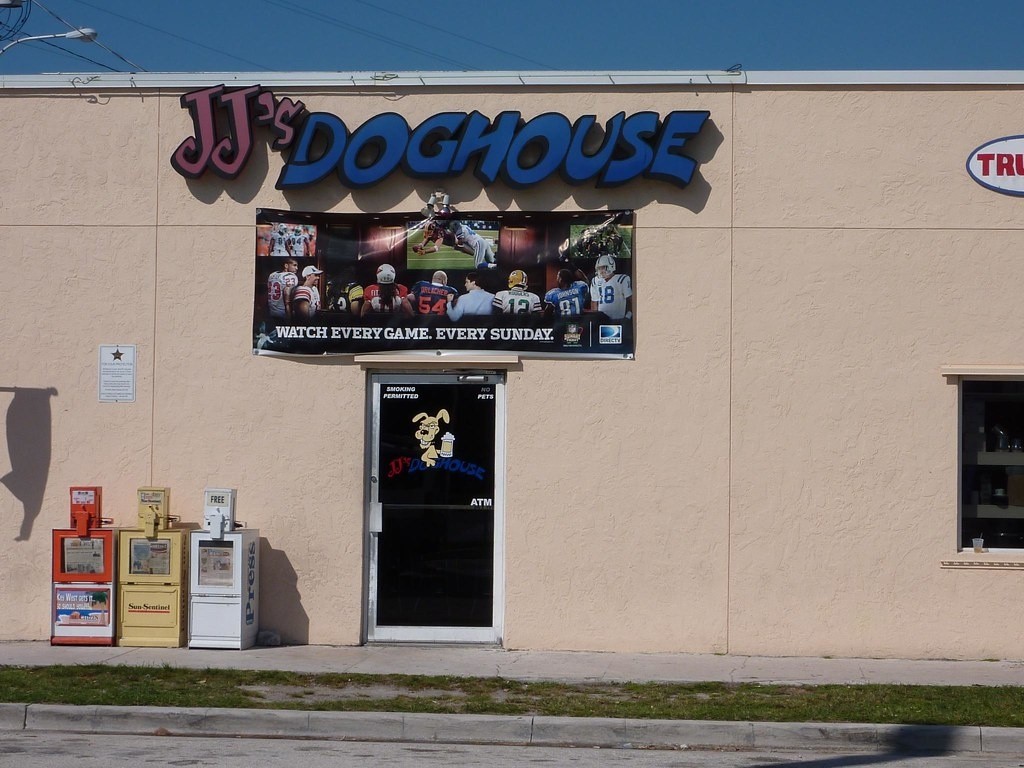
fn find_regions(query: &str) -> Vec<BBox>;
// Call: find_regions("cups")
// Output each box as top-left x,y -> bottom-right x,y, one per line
972,538 -> 984,553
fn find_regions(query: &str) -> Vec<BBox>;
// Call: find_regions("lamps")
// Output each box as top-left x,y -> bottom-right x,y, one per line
421,189 -> 451,219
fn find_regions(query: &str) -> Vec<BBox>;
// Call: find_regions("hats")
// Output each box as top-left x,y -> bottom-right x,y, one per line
302,266 -> 324,278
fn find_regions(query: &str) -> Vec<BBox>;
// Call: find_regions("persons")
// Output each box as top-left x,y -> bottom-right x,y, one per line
257,220 -> 634,323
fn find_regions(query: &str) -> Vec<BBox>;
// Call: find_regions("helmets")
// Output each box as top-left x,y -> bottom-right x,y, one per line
294,226 -> 301,235
508,270 -> 528,290
278,224 -> 288,234
376,264 -> 395,284
595,255 -> 616,276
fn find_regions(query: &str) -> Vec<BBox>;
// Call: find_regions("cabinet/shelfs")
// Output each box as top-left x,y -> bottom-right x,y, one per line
961,450 -> 1024,519
